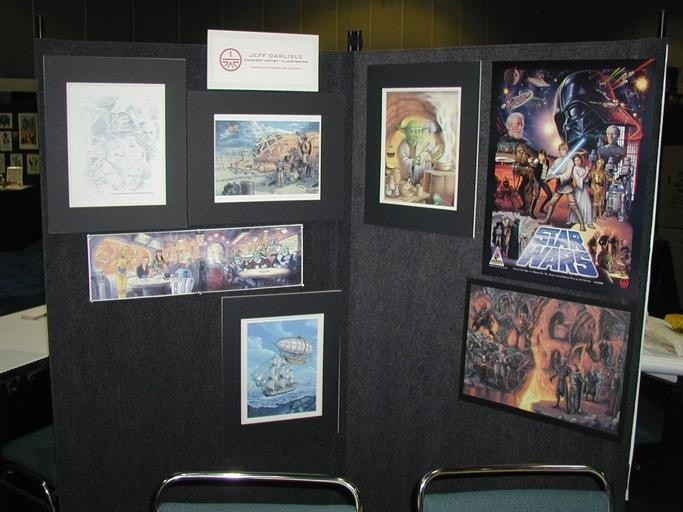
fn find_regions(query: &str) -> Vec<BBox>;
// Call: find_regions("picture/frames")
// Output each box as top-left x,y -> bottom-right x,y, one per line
480,56 -> 658,299
221,290 -> 344,439
190,88 -> 347,225
457,278 -> 633,445
44,57 -> 188,236
0,111 -> 42,191
363,62 -> 484,240
86,222 -> 306,304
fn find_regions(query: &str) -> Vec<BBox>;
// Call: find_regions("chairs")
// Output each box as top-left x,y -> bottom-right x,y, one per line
417,465 -> 608,512
151,471 -> 360,512
0,453 -> 59,512
